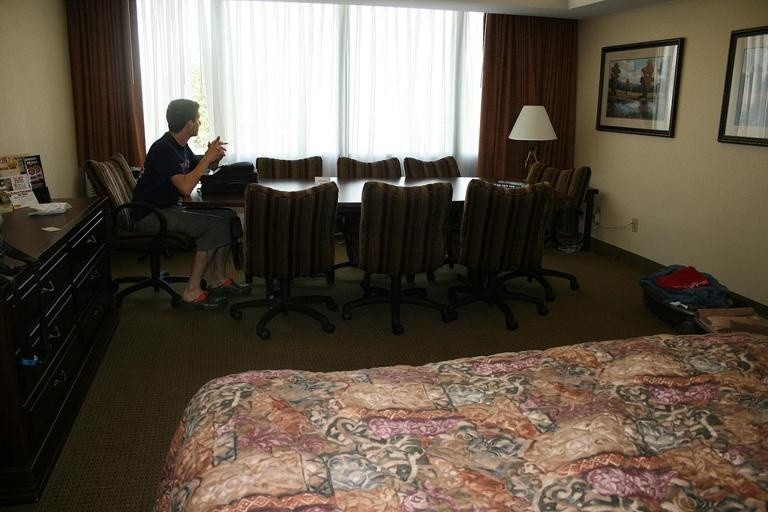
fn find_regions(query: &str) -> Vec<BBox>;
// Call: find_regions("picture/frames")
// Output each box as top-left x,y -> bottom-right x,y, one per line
595,38 -> 683,138
717,25 -> 768,146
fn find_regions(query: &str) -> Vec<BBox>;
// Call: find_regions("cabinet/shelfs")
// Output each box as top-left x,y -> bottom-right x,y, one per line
0,188 -> 117,504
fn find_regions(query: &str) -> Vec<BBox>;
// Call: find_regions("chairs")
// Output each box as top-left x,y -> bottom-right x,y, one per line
328,157 -> 399,289
342,181 -> 449,333
254,156 -> 324,184
442,181 -> 550,330
529,162 -> 592,255
83,153 -> 207,310
402,155 -> 460,284
230,181 -> 338,339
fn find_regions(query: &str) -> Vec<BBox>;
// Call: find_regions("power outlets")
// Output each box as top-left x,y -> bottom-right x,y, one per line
630,219 -> 639,233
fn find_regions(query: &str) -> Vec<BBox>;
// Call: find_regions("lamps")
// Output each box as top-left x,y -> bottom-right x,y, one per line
508,105 -> 556,179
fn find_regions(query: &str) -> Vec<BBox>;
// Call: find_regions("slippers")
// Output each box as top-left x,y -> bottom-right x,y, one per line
179,292 -> 226,312
207,279 -> 251,298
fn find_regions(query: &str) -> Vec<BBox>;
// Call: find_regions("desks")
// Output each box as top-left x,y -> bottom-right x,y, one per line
182,168 -> 529,288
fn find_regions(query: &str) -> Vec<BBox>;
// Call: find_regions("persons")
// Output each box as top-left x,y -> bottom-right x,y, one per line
130,102 -> 252,308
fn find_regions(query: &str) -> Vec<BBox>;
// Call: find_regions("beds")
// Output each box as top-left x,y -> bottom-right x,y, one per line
160,330 -> 764,512
